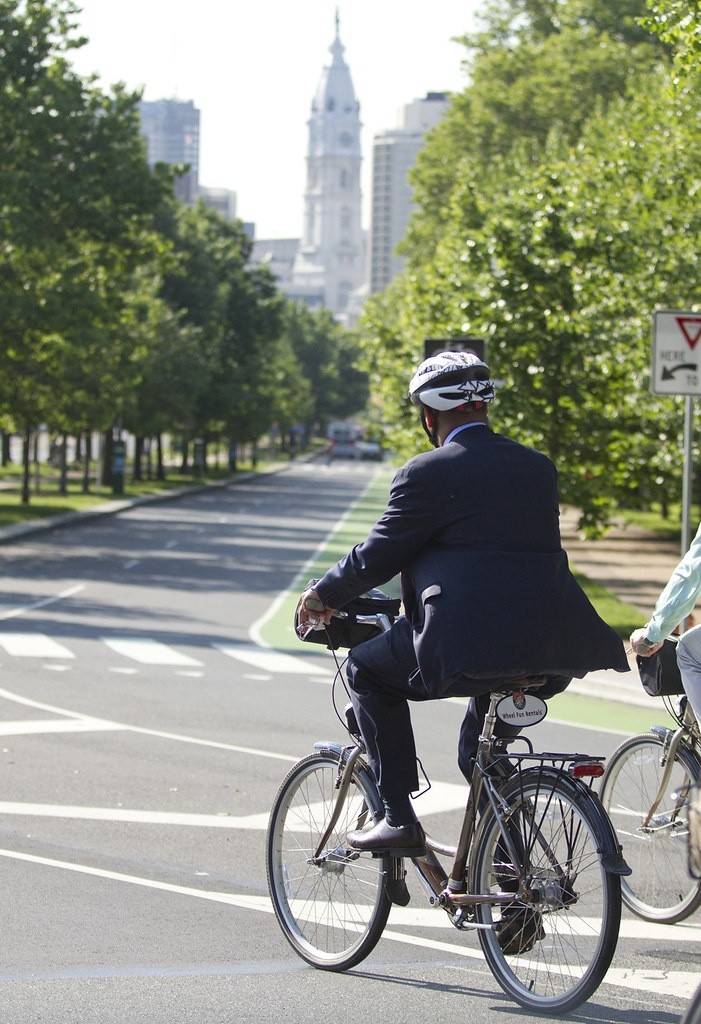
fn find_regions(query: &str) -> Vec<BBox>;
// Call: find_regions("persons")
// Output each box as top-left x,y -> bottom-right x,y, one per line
297,351 -> 631,955
630,521 -> 701,742
287,436 -> 297,461
250,445 -> 258,467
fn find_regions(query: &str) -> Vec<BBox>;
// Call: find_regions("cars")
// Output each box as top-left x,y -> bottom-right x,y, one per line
324,423 -> 384,462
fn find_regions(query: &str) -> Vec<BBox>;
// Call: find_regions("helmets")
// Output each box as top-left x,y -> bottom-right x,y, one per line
409,352 -> 497,413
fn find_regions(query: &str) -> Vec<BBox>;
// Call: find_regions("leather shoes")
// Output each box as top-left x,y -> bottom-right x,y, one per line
347,818 -> 427,857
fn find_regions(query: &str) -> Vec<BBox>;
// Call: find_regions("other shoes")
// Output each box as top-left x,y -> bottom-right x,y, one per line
498,910 -> 546,956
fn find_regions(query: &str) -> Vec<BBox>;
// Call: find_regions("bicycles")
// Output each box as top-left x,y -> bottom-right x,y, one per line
264,598 -> 634,1015
598,637 -> 701,925
